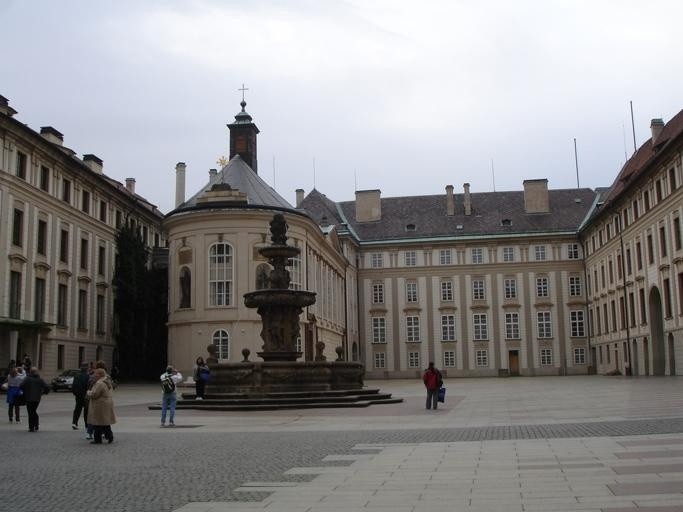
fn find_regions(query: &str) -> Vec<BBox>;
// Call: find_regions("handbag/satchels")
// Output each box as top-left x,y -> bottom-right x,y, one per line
2,383 -> 8,390
439,379 -> 443,386
200,370 -> 208,381
13,395 -> 25,406
437,388 -> 445,403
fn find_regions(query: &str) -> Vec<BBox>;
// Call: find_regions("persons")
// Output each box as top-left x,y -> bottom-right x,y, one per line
16,352 -> 32,378
18,366 -> 50,432
5,359 -> 16,377
191,356 -> 210,400
2,366 -> 27,423
84,367 -> 117,444
421,361 -> 442,410
81,358 -> 116,439
70,361 -> 90,430
157,364 -> 184,427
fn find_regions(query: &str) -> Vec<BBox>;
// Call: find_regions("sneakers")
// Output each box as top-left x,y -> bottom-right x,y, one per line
72,424 -> 79,429
9,417 -> 20,421
87,433 -> 113,444
28,425 -> 38,432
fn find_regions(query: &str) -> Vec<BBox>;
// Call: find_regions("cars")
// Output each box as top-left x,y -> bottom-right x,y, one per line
51,368 -> 81,392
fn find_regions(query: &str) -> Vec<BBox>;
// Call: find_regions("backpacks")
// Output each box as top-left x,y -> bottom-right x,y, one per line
161,375 -> 175,393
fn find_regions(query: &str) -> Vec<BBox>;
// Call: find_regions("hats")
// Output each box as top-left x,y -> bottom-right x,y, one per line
81,362 -> 89,369
167,366 -> 172,370
